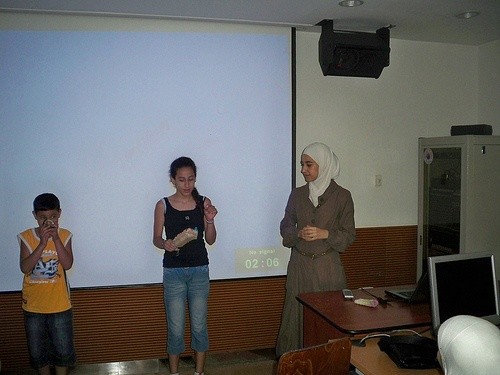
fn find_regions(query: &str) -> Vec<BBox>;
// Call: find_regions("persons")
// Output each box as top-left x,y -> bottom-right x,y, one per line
276,143 -> 357,360
152,156 -> 217,375
17,193 -> 77,375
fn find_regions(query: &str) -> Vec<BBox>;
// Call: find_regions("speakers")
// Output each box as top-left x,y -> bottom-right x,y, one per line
319,31 -> 388,79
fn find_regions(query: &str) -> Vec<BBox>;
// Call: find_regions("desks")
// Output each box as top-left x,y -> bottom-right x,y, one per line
329,327 -> 445,375
295,285 -> 433,348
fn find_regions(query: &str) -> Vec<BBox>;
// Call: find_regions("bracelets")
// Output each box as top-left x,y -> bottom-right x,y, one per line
206,219 -> 214,224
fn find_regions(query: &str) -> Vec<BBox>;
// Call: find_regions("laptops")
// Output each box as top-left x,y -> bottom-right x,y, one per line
428,252 -> 500,336
385,272 -> 430,304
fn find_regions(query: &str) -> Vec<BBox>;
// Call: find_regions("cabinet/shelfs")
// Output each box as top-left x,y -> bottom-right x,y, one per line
418,135 -> 500,282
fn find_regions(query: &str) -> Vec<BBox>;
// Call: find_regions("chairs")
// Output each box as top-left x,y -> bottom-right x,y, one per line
277,337 -> 352,375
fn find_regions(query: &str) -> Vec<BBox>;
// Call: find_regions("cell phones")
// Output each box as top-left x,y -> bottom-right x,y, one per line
343,288 -> 354,299
